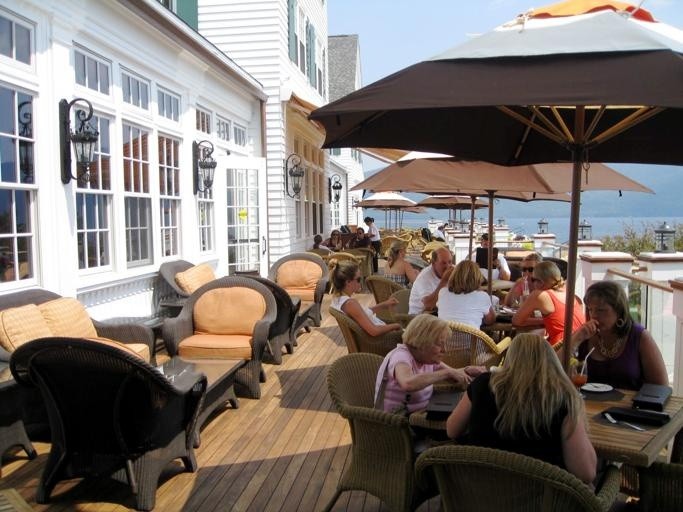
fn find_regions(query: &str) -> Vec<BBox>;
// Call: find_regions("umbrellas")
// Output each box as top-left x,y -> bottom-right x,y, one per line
351,191 -> 490,233
306,0 -> 683,381
346,152 -> 656,308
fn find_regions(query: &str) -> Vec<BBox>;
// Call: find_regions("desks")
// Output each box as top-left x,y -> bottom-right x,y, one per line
409,381 -> 682,467
295,302 -> 314,346
157,356 -> 246,446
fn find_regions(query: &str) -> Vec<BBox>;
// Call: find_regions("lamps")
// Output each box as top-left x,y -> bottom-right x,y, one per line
59,97 -> 96,189
329,172 -> 343,205
9,99 -> 31,184
191,139 -> 215,198
285,151 -> 305,201
478,215 -> 549,234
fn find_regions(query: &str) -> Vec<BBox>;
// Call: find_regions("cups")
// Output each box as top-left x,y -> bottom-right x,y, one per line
523,280 -> 530,296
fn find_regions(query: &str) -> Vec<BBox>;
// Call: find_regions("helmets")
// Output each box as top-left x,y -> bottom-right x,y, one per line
521,266 -> 533,272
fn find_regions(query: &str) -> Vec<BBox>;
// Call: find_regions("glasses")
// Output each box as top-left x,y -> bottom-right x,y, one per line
353,277 -> 361,283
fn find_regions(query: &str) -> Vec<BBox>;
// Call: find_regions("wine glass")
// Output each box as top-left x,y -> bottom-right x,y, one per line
571,361 -> 588,399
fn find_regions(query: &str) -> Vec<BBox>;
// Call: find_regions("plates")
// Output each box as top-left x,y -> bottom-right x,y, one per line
580,382 -> 614,393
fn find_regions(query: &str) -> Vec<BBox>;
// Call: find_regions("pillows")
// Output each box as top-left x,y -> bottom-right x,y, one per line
38,296 -> 98,338
3,305 -> 52,349
174,262 -> 216,296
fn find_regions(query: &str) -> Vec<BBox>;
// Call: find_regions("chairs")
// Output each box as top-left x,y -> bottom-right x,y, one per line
245,275 -> 302,363
9,338 -> 207,510
323,353 -> 446,511
416,444 -> 621,512
624,429 -> 683,510
164,276 -> 279,401
324,225 -> 577,370
159,260 -> 218,310
268,253 -> 329,327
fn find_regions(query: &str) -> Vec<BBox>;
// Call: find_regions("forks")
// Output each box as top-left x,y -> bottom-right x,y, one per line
605,412 -> 645,431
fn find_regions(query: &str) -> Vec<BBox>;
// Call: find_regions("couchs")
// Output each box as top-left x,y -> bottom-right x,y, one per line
1,289 -> 159,460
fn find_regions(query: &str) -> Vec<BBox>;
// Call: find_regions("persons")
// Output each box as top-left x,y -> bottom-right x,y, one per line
465,234 -> 511,282
407,245 -> 587,345
373,313 -> 489,465
328,258 -> 405,336
447,332 -> 597,491
314,216 -> 382,273
555,281 -> 667,391
386,239 -> 419,289
